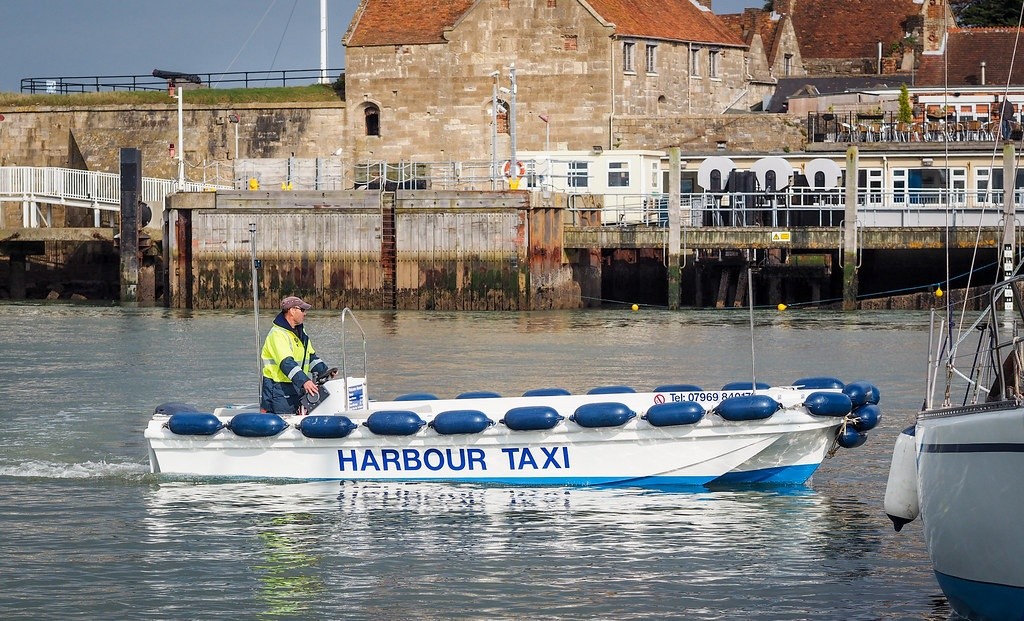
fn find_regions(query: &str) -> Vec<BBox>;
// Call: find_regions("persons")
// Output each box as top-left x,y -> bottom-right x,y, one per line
260,297 -> 338,415
999,95 -> 1014,140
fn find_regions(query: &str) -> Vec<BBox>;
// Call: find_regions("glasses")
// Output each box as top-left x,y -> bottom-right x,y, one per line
296,307 -> 306,312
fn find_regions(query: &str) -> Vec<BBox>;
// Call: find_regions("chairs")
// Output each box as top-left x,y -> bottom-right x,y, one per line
823,118 -> 1024,141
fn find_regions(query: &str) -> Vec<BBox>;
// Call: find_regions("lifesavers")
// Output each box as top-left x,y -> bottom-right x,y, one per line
500,158 -> 526,184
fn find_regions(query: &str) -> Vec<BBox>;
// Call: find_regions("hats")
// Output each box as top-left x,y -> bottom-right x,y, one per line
281,296 -> 311,311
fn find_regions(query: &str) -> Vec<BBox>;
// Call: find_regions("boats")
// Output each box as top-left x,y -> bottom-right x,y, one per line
143,222 -> 880,486
885,0 -> 1024,621
